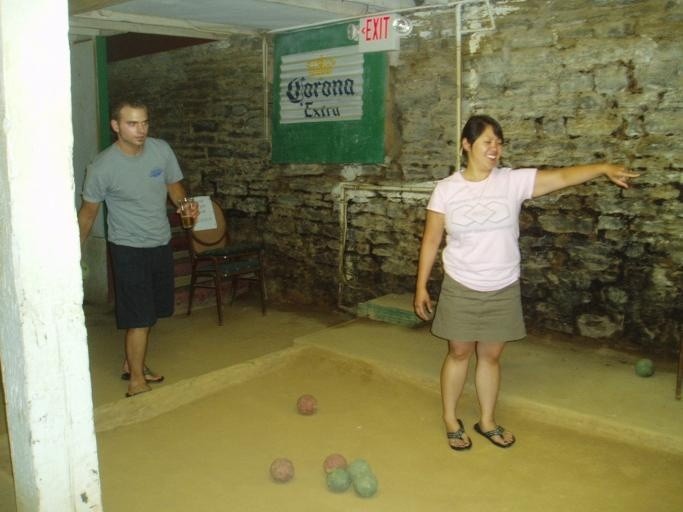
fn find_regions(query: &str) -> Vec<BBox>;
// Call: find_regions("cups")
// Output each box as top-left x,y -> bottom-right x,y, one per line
178,196 -> 195,229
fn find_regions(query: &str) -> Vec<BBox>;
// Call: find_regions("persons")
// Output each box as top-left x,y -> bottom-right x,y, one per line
79,97 -> 200,399
413,115 -> 640,452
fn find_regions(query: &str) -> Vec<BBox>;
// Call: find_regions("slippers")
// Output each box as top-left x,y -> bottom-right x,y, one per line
447,418 -> 472,450
121,368 -> 163,382
474,421 -> 516,448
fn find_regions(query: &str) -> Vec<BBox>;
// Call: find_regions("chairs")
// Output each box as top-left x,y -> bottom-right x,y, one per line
182,196 -> 267,326
